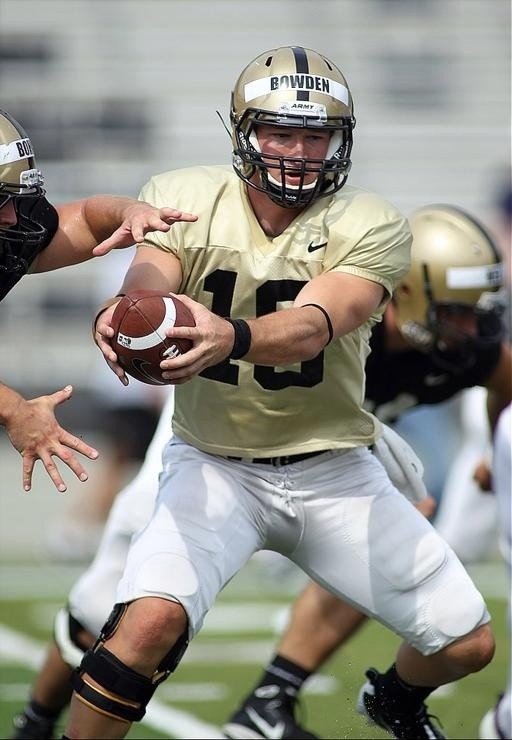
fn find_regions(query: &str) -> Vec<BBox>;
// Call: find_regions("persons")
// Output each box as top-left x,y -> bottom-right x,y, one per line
434,183 -> 512,739
0,106 -> 202,492
8,201 -> 511,740
51,42 -> 499,739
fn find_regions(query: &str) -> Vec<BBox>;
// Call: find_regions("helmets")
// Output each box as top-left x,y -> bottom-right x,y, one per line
0,107 -> 39,204
390,203 -> 503,347
229,47 -> 356,175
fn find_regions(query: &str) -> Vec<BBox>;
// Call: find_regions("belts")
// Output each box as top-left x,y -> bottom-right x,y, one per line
227,448 -> 334,468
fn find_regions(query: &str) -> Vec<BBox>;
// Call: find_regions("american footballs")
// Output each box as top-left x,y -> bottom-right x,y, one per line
106,289 -> 197,385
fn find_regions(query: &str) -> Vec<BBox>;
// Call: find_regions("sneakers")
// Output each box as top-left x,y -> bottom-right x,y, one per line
355,666 -> 448,739
477,693 -> 509,740
221,697 -> 320,739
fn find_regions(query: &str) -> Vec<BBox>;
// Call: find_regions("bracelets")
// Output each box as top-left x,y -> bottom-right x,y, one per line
222,316 -> 252,360
